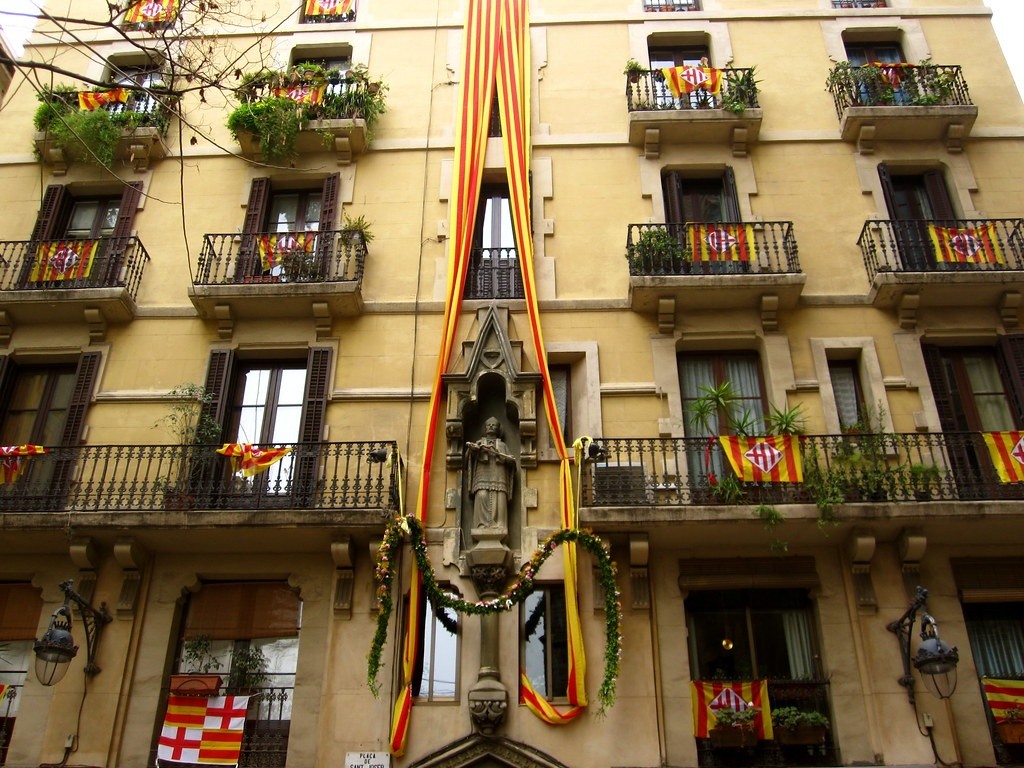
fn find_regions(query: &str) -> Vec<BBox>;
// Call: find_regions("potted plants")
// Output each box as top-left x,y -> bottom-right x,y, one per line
694,388 -> 939,505
170,633 -> 224,694
708,706 -> 830,748
341,214 -> 376,249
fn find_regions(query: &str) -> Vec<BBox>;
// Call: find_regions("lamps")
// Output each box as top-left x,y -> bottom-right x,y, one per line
34,580 -> 113,687
888,585 -> 960,704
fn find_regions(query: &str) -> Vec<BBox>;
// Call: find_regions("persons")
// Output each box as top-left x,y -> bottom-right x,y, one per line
463,416 -> 515,529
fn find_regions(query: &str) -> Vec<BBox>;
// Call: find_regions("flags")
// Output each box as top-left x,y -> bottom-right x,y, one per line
983,678 -> 1024,744
277,83 -> 328,106
303,0 -> 353,16
719,434 -> 804,483
981,431 -> 1024,482
0,446 -> 44,482
665,66 -> 723,97
125,0 -> 179,22
928,224 -> 1004,264
78,88 -> 129,113
257,233 -> 316,270
863,62 -> 918,87
155,694 -> 249,766
691,679 -> 773,740
685,222 -> 755,261
218,444 -> 293,478
30,240 -> 97,279
